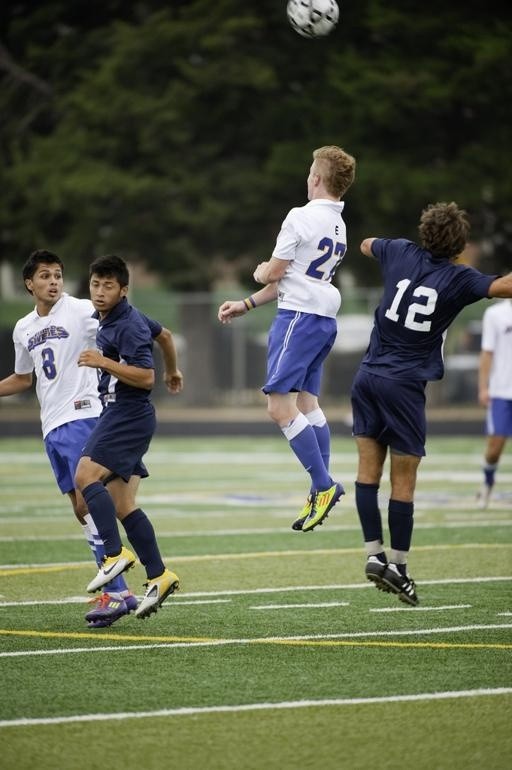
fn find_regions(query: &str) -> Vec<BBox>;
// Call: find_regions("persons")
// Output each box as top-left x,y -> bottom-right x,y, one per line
2,248 -> 139,628
217,147 -> 358,531
75,254 -> 185,621
350,201 -> 511,605
476,297 -> 509,513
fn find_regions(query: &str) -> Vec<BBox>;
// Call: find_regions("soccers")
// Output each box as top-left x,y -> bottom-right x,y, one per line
286,0 -> 339,39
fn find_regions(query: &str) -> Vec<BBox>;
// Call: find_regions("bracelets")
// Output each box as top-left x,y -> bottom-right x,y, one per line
241,295 -> 256,311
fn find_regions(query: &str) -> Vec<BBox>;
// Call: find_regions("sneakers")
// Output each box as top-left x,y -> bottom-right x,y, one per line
87,547 -> 136,593
134,568 -> 180,620
85,590 -> 137,628
291,482 -> 345,532
479,480 -> 494,509
365,555 -> 417,607
